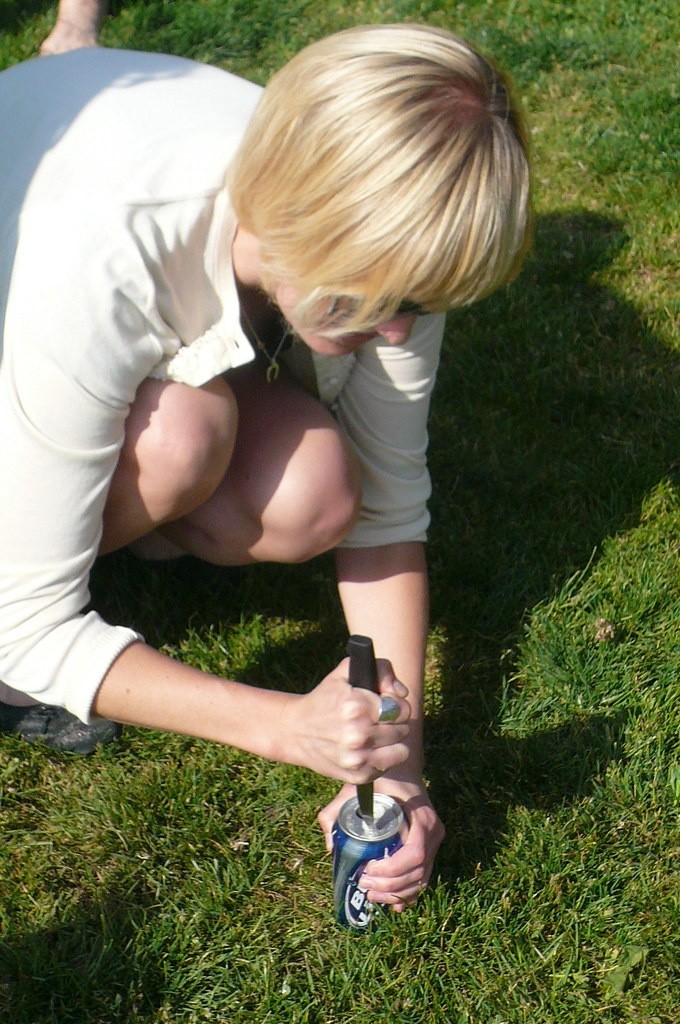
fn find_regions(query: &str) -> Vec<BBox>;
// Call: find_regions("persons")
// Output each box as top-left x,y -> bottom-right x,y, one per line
1,22 -> 538,915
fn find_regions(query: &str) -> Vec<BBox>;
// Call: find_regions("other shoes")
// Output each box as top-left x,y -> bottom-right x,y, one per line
0,703 -> 118,754
115,546 -> 204,579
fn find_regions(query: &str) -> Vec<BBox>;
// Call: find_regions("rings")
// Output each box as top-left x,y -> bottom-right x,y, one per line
376,697 -> 401,722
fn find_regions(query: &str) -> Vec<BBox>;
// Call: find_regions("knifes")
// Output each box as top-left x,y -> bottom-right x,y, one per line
344,634 -> 377,825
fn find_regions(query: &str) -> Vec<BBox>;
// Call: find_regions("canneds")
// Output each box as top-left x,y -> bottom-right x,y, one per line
331,792 -> 409,933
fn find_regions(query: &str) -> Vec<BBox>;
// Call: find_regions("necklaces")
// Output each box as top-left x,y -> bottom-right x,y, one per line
242,305 -> 292,385
